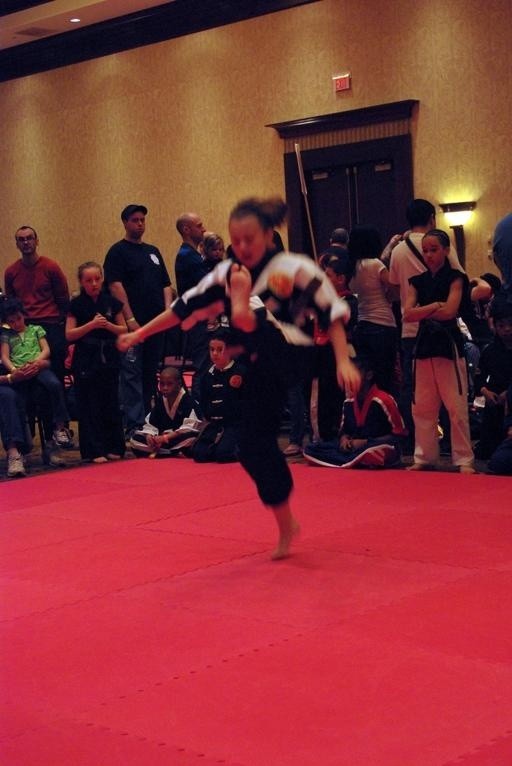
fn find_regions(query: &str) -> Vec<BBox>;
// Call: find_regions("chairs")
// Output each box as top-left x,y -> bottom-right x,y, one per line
42,426 -> 79,467
6,453 -> 26,478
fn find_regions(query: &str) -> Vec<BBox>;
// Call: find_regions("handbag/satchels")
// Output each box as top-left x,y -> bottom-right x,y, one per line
125,317 -> 136,324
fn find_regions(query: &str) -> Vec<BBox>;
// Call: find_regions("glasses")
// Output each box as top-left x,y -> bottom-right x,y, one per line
434,199 -> 478,273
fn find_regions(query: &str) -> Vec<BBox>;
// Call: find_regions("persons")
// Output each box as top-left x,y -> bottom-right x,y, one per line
344,226 -> 399,396
198,230 -> 230,332
281,369 -> 310,458
174,212 -> 214,422
102,203 -> 173,460
1,290 -> 77,479
113,190 -> 367,563
63,259 -> 131,465
387,196 -> 512,478
315,253 -> 361,442
129,366 -> 203,461
316,226 -> 357,258
3,226 -> 75,451
190,331 -> 235,466
301,353 -> 411,469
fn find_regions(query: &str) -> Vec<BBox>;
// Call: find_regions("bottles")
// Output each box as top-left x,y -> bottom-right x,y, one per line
121,205 -> 147,220
